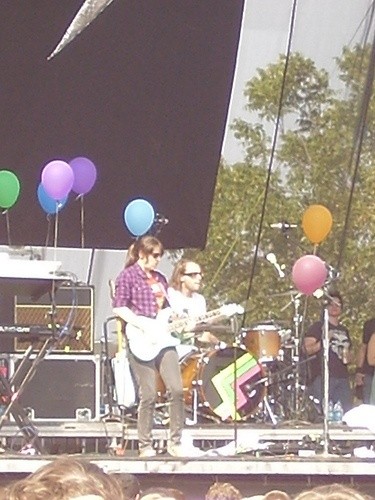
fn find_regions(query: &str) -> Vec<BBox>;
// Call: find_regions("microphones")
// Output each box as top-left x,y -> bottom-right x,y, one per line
267,252 -> 285,278
155,218 -> 168,224
270,223 -> 297,228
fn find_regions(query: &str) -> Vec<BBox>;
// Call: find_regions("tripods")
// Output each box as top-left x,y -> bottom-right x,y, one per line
271,294 -> 324,427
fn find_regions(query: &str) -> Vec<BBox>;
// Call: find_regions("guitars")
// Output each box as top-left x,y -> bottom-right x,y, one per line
108,279 -> 136,409
124,303 -> 245,362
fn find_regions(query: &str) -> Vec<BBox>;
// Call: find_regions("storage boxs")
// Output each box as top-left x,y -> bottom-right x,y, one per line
10,353 -> 102,421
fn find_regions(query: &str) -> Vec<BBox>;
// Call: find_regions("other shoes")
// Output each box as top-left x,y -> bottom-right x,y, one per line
140,449 -> 156,457
167,445 -> 186,457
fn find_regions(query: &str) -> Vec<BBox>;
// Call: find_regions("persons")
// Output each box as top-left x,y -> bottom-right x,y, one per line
0,459 -> 375,500
355,317 -> 375,406
113,234 -> 187,458
301,289 -> 353,413
166,258 -> 226,357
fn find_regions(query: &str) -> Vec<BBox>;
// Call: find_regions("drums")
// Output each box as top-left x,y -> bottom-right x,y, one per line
239,324 -> 282,364
179,345 -> 267,423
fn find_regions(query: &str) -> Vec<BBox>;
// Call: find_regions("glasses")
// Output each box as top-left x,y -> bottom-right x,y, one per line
150,253 -> 163,258
183,272 -> 202,279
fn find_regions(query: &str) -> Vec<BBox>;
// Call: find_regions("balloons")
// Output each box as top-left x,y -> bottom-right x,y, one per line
41,160 -> 73,199
69,157 -> 97,194
124,198 -> 155,237
303,204 -> 332,243
292,255 -> 327,296
0,170 -> 20,209
37,183 -> 67,215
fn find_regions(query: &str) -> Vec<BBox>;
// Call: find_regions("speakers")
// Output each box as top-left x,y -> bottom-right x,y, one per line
14,285 -> 95,357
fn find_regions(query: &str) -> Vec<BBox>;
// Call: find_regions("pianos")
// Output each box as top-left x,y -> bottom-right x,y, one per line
0,324 -> 88,455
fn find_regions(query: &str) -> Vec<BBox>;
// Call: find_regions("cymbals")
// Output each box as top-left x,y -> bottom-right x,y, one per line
269,290 -> 302,298
254,318 -> 289,323
